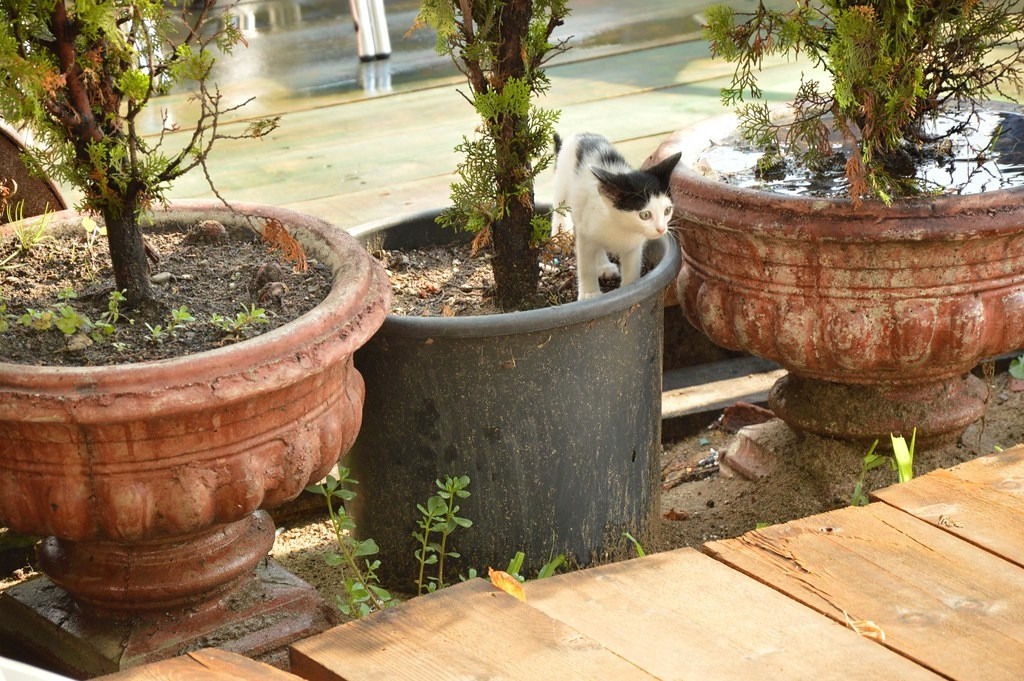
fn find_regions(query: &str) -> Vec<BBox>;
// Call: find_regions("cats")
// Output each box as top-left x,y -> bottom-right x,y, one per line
552,131 -> 683,301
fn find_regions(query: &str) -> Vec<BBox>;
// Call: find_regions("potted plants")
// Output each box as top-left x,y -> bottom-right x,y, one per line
642,0 -> 1024,454
335,0 -> 682,603
0,0 -> 392,674
